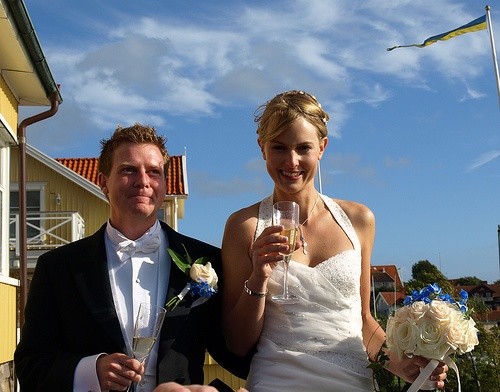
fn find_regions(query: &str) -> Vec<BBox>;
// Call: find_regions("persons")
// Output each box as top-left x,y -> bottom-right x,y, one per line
219,89 -> 450,392
14,124 -> 255,392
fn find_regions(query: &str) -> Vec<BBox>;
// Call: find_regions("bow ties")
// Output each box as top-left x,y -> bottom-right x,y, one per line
106,219 -> 161,262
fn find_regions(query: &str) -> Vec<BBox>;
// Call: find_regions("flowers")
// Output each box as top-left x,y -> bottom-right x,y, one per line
382,280 -> 480,392
168,246 -> 222,316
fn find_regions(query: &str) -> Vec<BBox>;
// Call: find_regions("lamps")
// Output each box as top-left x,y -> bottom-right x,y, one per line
51,191 -> 62,207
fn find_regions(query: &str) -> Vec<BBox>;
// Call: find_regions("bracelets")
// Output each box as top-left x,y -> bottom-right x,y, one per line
243,279 -> 269,296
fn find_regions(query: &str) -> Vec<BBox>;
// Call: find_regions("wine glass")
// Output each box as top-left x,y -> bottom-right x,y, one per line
125,303 -> 167,392
271,201 -> 300,304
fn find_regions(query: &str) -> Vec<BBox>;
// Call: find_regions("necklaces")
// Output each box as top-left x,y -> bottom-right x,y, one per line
271,192 -> 319,256
